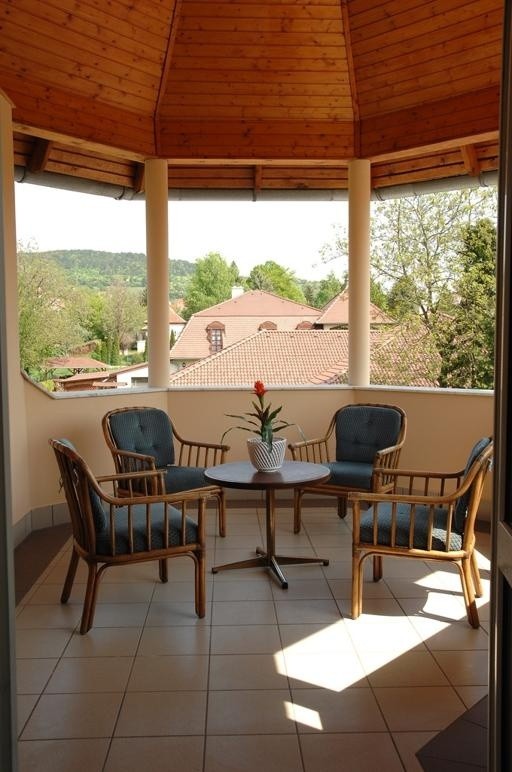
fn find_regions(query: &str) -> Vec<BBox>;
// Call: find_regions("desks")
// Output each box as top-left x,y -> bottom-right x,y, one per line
202,460 -> 332,590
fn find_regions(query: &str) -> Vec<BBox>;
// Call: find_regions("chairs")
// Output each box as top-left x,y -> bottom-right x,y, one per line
48,436 -> 223,635
287,403 -> 408,535
347,433 -> 493,629
101,406 -> 231,537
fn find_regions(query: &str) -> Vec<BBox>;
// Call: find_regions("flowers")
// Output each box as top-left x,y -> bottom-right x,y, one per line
219,380 -> 296,454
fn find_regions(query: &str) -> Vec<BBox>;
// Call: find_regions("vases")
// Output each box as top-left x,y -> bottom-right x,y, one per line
247,436 -> 287,474
